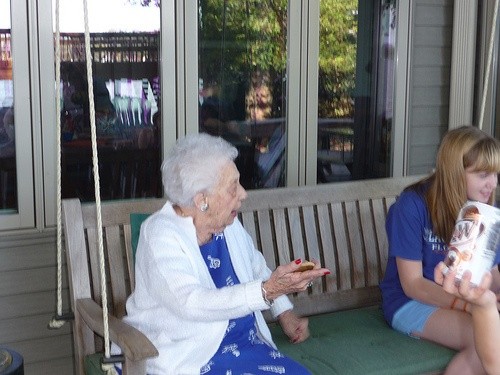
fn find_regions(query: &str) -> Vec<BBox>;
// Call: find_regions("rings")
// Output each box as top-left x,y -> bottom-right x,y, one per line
307,281 -> 314,287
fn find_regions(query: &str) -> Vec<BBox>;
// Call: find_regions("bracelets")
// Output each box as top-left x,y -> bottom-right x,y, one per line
451,296 -> 458,310
262,286 -> 274,306
462,301 -> 471,313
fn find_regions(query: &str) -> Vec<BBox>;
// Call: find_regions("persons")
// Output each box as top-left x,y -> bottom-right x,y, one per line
434,261 -> 500,375
110,132 -> 333,374
379,126 -> 499,375
